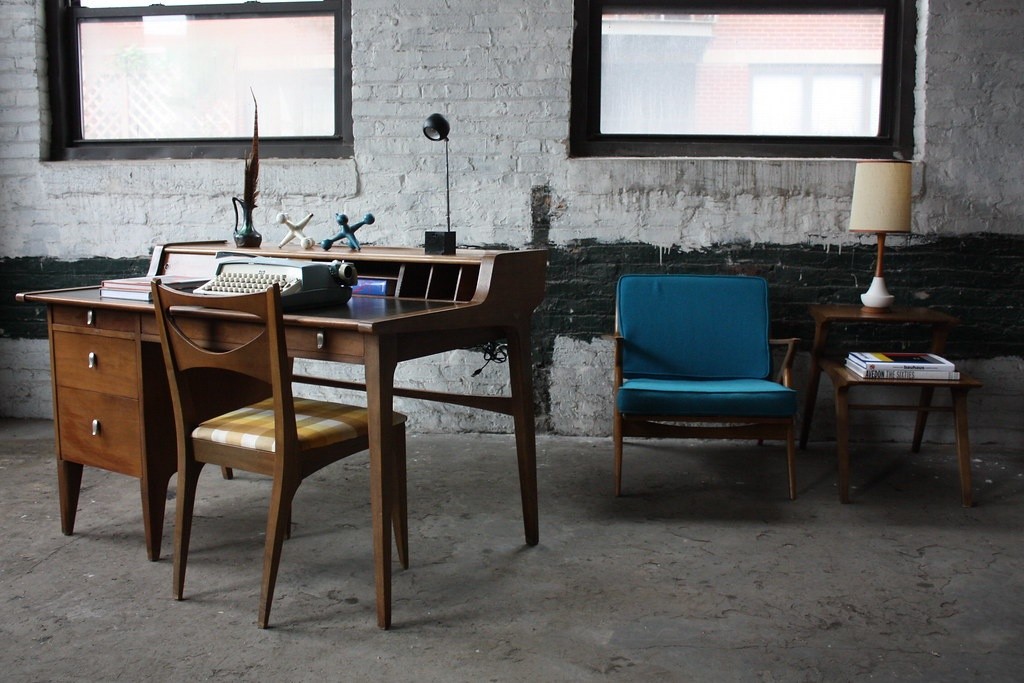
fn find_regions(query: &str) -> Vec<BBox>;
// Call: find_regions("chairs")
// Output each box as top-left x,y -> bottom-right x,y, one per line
150,278 -> 413,632
611,272 -> 797,500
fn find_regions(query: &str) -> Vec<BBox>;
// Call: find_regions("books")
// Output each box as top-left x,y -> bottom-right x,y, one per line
99,275 -> 210,301
845,352 -> 960,379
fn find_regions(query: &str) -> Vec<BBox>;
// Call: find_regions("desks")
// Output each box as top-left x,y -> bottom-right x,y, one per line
13,234 -> 556,630
799,301 -> 983,507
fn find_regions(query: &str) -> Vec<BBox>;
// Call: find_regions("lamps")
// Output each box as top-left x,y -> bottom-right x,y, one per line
422,112 -> 455,231
846,158 -> 914,306
